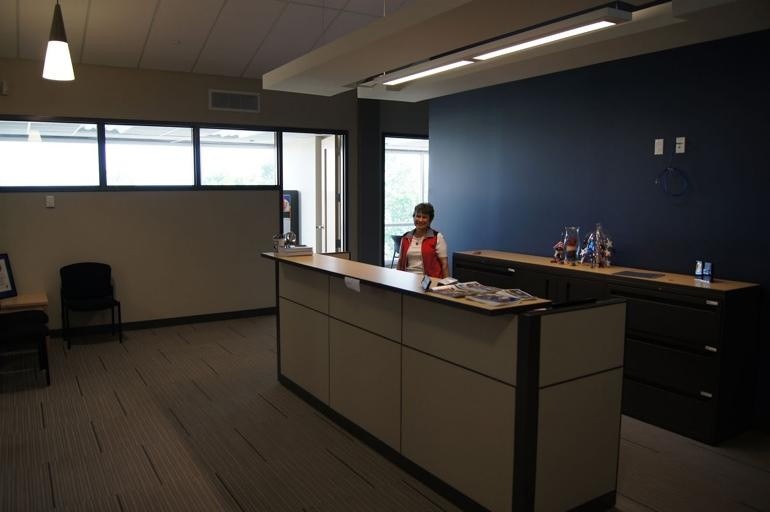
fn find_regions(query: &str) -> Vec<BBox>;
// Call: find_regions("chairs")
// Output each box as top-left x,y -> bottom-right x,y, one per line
0,310 -> 51,387
390,236 -> 405,269
58,262 -> 122,350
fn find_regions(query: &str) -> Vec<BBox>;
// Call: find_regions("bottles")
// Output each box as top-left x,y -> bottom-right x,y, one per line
563,226 -> 581,265
593,222 -> 606,264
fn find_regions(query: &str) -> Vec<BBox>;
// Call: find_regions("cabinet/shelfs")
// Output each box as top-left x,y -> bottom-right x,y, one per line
452,252 -> 761,447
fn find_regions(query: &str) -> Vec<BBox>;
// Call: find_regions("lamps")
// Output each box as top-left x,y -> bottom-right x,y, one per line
382,1 -> 632,88
41,1 -> 77,83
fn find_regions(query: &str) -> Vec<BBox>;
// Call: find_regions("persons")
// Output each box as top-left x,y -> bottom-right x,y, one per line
396,202 -> 448,279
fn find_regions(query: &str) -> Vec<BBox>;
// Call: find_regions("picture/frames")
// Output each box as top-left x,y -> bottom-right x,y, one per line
0,253 -> 18,300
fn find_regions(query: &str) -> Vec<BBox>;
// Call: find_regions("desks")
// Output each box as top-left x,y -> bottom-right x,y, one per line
0,291 -> 49,358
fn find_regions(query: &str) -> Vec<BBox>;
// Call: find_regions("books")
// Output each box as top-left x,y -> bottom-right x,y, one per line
277,245 -> 313,254
465,289 -> 537,306
431,281 -> 501,298
278,251 -> 313,257
